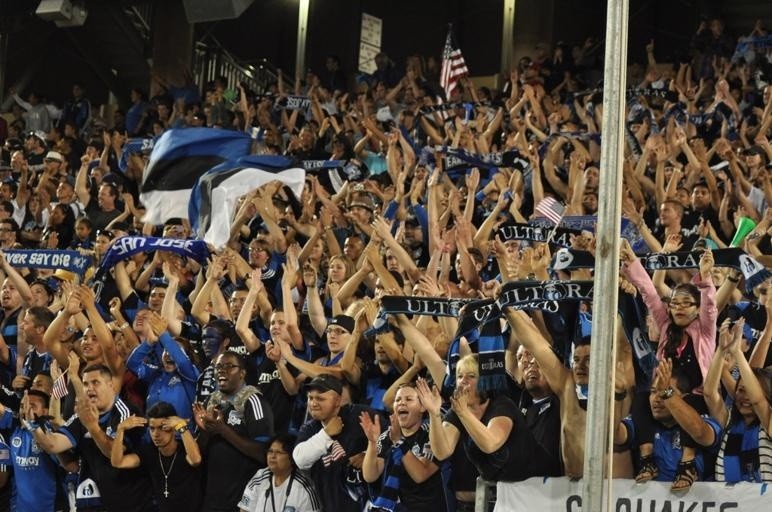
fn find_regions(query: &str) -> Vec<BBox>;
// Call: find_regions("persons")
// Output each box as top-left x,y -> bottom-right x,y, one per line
1,13 -> 771,511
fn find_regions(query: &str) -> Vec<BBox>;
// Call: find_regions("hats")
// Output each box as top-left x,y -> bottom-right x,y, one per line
305,375 -> 342,395
28,128 -> 47,145
347,195 -> 375,212
328,315 -> 354,334
44,151 -> 62,163
744,146 -> 765,156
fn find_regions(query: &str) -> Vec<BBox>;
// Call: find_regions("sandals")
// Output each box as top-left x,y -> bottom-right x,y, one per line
670,459 -> 698,491
634,455 -> 658,482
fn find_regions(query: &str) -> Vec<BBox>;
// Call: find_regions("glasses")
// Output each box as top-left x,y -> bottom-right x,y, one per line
324,329 -> 345,334
249,247 -> 266,252
213,363 -> 241,370
667,300 -> 697,307
